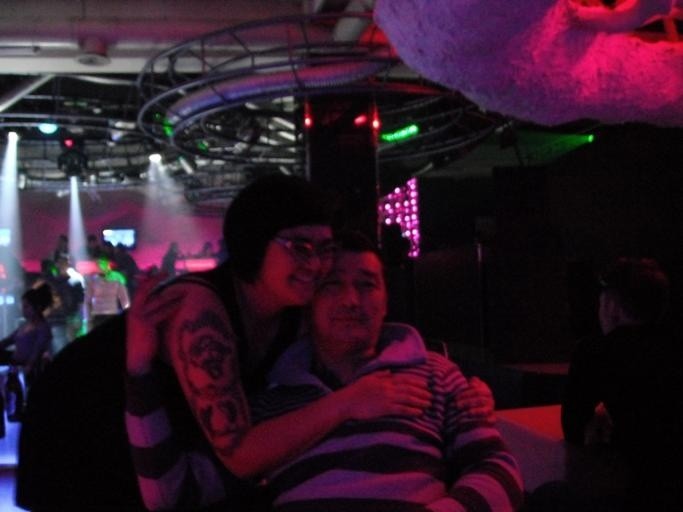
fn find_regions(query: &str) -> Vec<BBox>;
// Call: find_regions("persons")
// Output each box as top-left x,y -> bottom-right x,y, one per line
1,232 -> 230,439
15,173 -> 433,510
524,254 -> 682,509
237,235 -> 525,509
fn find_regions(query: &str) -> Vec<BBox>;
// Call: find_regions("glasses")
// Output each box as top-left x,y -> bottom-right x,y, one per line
270,234 -> 342,260
